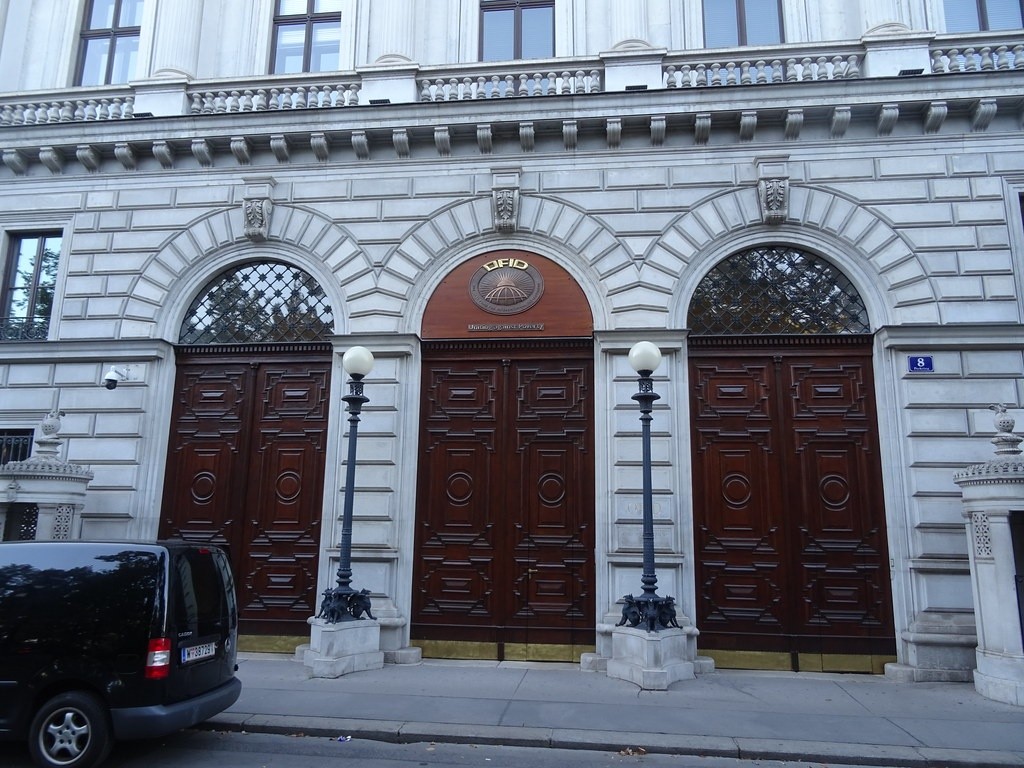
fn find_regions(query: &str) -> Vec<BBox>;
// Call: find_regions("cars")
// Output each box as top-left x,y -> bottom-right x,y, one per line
0,540 -> 242,767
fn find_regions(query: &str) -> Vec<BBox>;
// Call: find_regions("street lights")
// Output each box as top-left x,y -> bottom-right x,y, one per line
314,346 -> 380,626
615,337 -> 685,632
